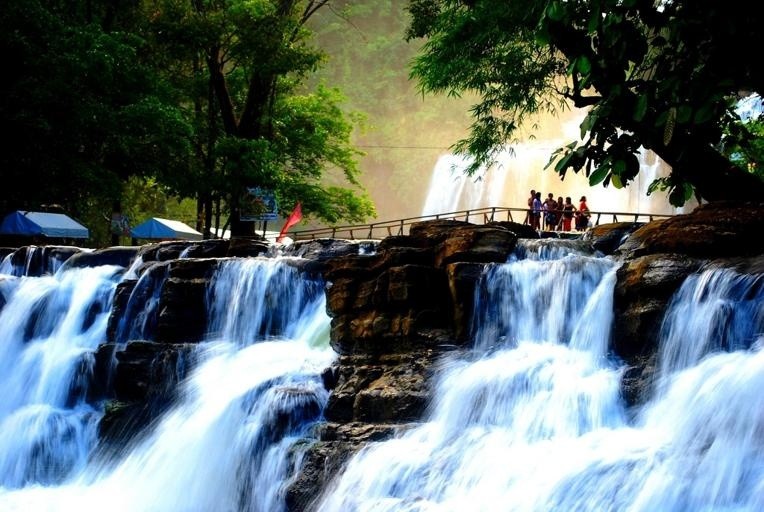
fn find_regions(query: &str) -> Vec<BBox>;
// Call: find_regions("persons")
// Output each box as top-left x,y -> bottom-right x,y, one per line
525,189 -> 591,232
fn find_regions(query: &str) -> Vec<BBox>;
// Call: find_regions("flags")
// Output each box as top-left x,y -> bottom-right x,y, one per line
277,205 -> 303,245
